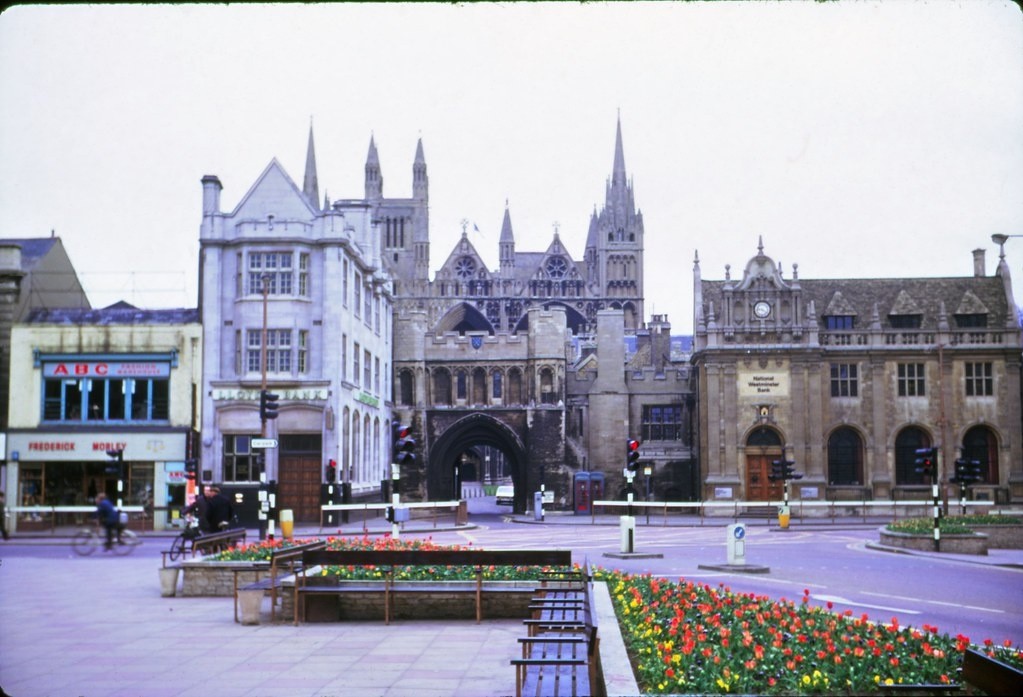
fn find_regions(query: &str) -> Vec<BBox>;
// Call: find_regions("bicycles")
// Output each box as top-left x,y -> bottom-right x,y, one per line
72,520 -> 143,555
169,514 -> 236,562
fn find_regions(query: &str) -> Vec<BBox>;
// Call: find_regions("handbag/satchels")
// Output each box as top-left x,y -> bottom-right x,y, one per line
224,503 -> 232,520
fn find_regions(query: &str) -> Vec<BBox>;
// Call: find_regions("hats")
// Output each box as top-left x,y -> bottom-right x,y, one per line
210,487 -> 220,491
98,492 -> 105,498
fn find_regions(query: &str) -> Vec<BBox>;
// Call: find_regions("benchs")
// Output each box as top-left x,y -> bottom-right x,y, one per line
294,550 -> 572,625
509,553 -> 599,697
232,540 -> 327,624
161,527 -> 246,565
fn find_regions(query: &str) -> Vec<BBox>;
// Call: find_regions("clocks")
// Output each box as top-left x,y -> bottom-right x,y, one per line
754,301 -> 772,318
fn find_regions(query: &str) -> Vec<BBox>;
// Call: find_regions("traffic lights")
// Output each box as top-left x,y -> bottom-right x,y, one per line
955,460 -> 980,482
260,389 -> 279,419
786,460 -> 803,480
769,461 -> 784,480
329,459 -> 336,482
185,458 -> 201,479
392,422 -> 415,464
106,450 -> 123,475
627,440 -> 640,471
915,447 -> 937,475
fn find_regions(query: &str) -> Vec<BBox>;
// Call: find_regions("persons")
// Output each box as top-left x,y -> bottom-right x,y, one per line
0,492 -> 10,540
181,485 -> 212,554
69,488 -> 83,525
207,487 -> 231,553
88,493 -> 119,550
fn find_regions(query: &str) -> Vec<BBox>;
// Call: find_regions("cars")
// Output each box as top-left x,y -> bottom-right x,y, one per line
496,486 -> 514,504
